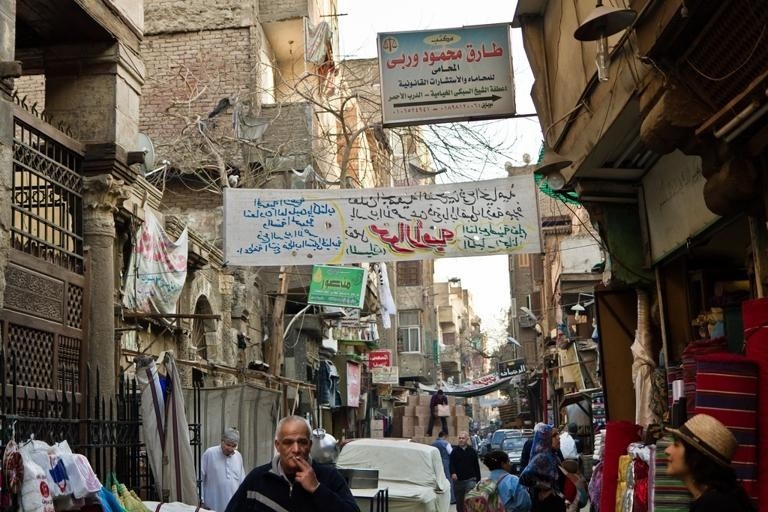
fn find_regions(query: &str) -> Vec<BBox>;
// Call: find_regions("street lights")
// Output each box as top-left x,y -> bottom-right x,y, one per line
519,304 -> 550,426
508,338 -> 538,357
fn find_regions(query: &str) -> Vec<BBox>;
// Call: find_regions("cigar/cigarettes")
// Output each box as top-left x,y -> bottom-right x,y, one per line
295,455 -> 301,460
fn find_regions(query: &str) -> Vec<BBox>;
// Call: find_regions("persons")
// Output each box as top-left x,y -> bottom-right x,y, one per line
520,422 -> 588,512
431,431 -> 457,504
449,431 -> 480,512
427,390 -> 450,436
200,426 -> 245,512
224,415 -> 360,512
483,451 -> 532,512
664,414 -> 758,512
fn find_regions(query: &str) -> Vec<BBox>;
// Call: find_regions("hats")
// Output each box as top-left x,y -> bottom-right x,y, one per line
551,427 -> 558,435
665,414 -> 738,466
222,426 -> 239,442
568,424 -> 577,435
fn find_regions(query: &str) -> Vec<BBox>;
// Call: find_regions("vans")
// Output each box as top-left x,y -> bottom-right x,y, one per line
491,427 -> 524,453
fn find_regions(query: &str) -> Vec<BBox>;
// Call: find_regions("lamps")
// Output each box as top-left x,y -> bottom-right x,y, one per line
572,0 -> 640,83
568,290 -> 597,321
280,306 -> 348,340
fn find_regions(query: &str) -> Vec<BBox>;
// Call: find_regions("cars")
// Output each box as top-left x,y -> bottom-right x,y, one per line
500,438 -> 530,470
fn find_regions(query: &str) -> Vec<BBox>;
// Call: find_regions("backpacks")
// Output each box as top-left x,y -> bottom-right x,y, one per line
464,472 -> 508,512
